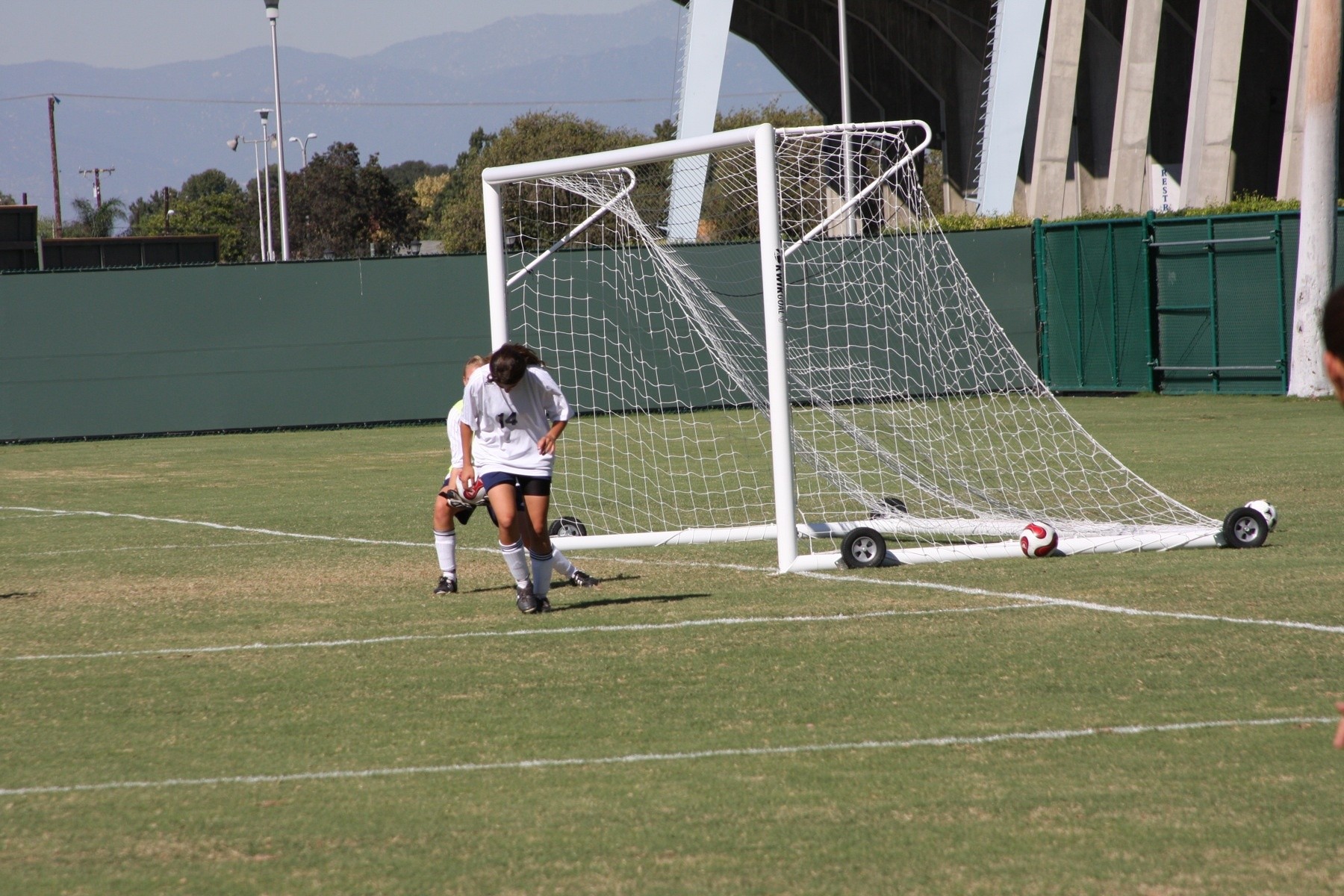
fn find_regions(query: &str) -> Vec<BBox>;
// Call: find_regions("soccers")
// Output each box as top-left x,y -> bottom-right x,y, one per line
1020,519 -> 1059,560
1245,498 -> 1277,530
456,470 -> 488,504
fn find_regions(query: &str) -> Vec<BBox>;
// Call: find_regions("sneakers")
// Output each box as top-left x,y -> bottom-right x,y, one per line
570,571 -> 598,589
514,580 -> 536,612
434,576 -> 457,594
534,595 -> 551,613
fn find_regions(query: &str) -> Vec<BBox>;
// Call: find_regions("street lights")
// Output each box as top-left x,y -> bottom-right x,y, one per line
254,107 -> 276,260
288,133 -> 318,233
262,0 -> 292,261
226,132 -> 279,262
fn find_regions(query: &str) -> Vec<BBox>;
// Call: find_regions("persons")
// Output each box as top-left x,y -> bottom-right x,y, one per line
1319,285 -> 1344,411
433,356 -> 598,595
459,343 -> 575,612
